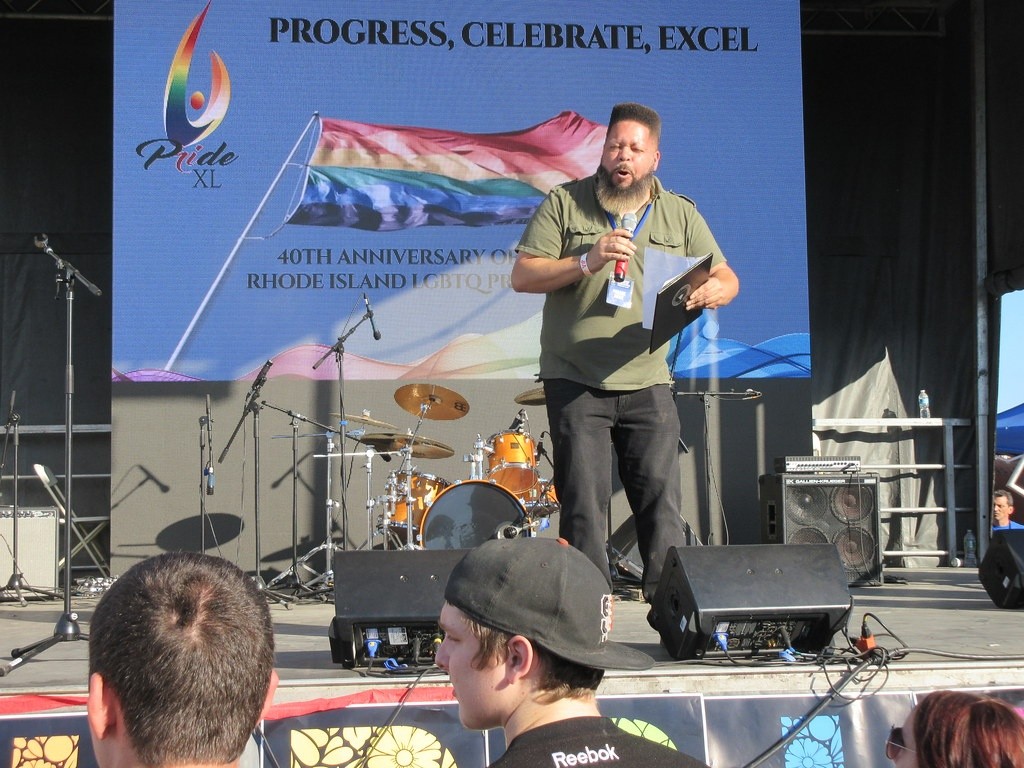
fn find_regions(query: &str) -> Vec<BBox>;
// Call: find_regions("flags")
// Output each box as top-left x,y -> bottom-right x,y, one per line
285,110 -> 609,228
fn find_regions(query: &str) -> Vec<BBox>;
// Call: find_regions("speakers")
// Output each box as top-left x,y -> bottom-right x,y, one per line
605,509 -> 707,582
978,529 -> 1024,610
650,544 -> 853,661
758,472 -> 884,585
0,504 -> 60,600
331,547 -> 473,670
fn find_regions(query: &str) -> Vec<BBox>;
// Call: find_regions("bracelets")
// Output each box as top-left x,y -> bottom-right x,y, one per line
580,252 -> 595,276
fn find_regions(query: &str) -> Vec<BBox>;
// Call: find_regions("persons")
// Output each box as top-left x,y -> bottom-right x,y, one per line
886,691 -> 1024,768
992,490 -> 1024,529
434,538 -> 708,768
512,103 -> 739,591
87,548 -> 278,768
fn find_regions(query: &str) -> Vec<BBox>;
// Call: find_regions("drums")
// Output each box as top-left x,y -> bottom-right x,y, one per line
377,431 -> 559,550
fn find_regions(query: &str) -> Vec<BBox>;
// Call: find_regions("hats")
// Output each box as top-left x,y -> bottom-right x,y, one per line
444,536 -> 655,671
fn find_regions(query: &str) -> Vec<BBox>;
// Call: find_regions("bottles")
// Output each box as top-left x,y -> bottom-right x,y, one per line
964,529 -> 978,568
919,389 -> 930,418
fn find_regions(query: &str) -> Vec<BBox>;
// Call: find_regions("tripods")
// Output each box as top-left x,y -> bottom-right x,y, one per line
217,378 -> 369,610
0,391 -> 65,608
0,233 -> 103,677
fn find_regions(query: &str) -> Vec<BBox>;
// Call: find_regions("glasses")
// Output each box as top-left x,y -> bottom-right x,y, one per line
886,728 -> 917,759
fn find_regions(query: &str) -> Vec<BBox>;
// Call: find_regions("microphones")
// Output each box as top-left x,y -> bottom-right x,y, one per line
363,293 -> 381,340
376,448 -> 391,463
503,526 -> 518,540
206,465 -> 214,496
245,360 -> 274,398
509,409 -> 524,430
614,213 -> 638,282
535,432 -> 545,461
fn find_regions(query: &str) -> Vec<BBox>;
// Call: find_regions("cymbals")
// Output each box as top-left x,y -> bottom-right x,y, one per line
393,383 -> 470,421
514,387 -> 545,406
328,412 -> 401,430
361,431 -> 455,459
